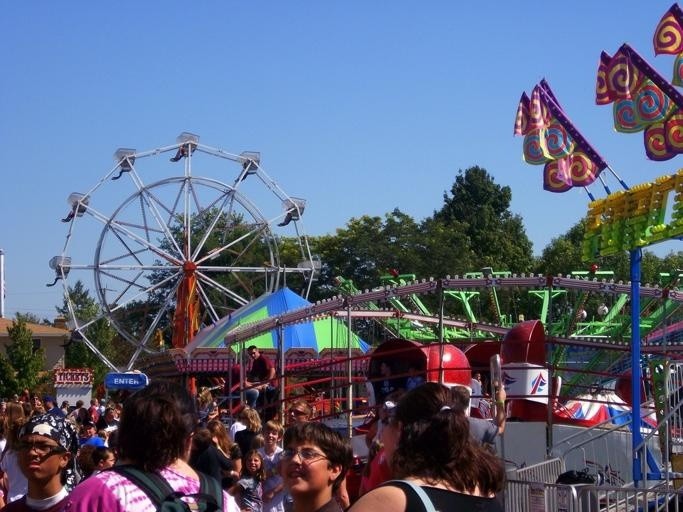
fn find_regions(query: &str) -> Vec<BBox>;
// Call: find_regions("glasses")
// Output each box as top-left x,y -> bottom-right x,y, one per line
277,447 -> 330,462
16,442 -> 59,456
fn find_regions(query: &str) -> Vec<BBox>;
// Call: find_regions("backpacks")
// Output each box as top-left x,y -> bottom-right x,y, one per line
101,464 -> 223,512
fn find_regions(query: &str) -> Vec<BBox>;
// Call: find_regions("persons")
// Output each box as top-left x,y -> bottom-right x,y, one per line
0,345 -> 509,511
